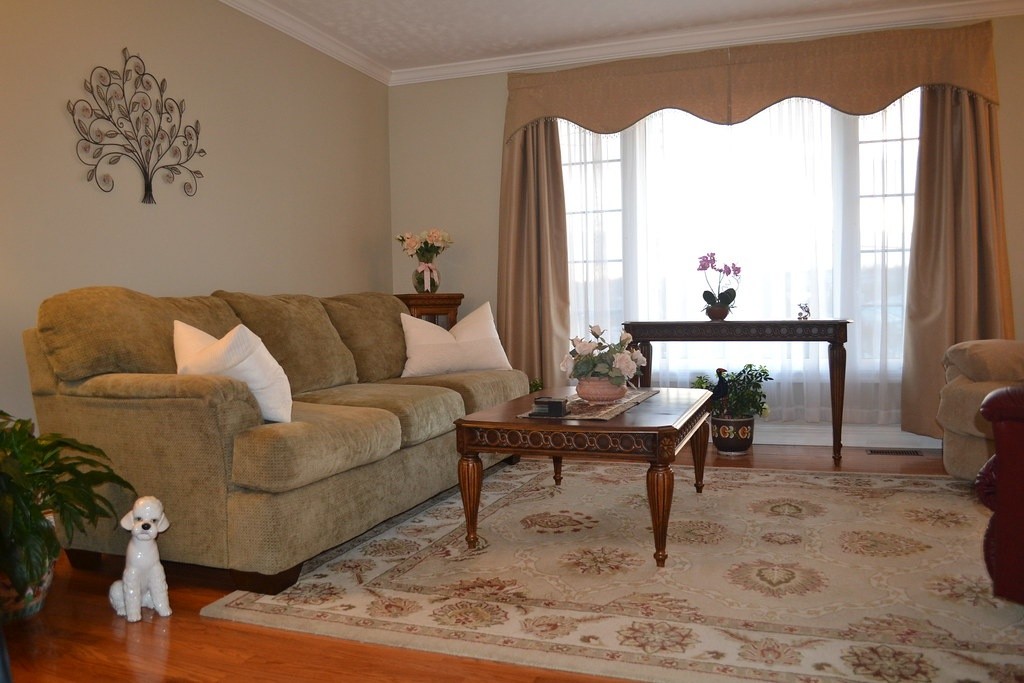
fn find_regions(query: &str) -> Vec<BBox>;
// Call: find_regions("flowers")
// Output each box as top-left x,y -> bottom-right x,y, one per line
559,324 -> 648,388
394,227 -> 455,286
689,363 -> 775,423
697,252 -> 742,315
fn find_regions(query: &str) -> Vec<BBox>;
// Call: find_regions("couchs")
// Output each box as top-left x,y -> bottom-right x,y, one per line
975,387 -> 1024,604
934,338 -> 1024,481
21,327 -> 530,594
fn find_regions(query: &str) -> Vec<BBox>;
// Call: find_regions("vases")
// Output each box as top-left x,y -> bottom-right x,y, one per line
711,414 -> 754,456
575,375 -> 628,407
411,251 -> 440,295
706,306 -> 730,321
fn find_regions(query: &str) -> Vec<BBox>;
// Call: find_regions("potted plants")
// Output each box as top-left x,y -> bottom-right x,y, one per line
0,409 -> 141,621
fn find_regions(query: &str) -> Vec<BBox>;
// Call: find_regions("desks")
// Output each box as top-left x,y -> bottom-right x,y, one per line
623,319 -> 855,468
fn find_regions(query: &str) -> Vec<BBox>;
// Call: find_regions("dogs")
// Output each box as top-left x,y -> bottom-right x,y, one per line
107,495 -> 173,622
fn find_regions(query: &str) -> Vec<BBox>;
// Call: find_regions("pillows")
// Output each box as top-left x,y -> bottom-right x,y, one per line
211,290 -> 359,395
316,292 -> 412,383
173,318 -> 293,425
40,285 -> 243,382
400,301 -> 513,378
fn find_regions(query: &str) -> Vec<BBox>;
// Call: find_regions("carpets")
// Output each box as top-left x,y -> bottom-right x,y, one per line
198,456 -> 1024,683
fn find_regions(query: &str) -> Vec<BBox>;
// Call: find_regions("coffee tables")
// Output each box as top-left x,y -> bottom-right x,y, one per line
452,384 -> 714,567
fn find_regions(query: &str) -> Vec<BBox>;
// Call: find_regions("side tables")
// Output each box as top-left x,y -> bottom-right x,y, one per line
394,293 -> 464,331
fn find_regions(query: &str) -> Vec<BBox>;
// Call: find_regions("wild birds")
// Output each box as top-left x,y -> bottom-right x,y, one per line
710,368 -> 729,406
